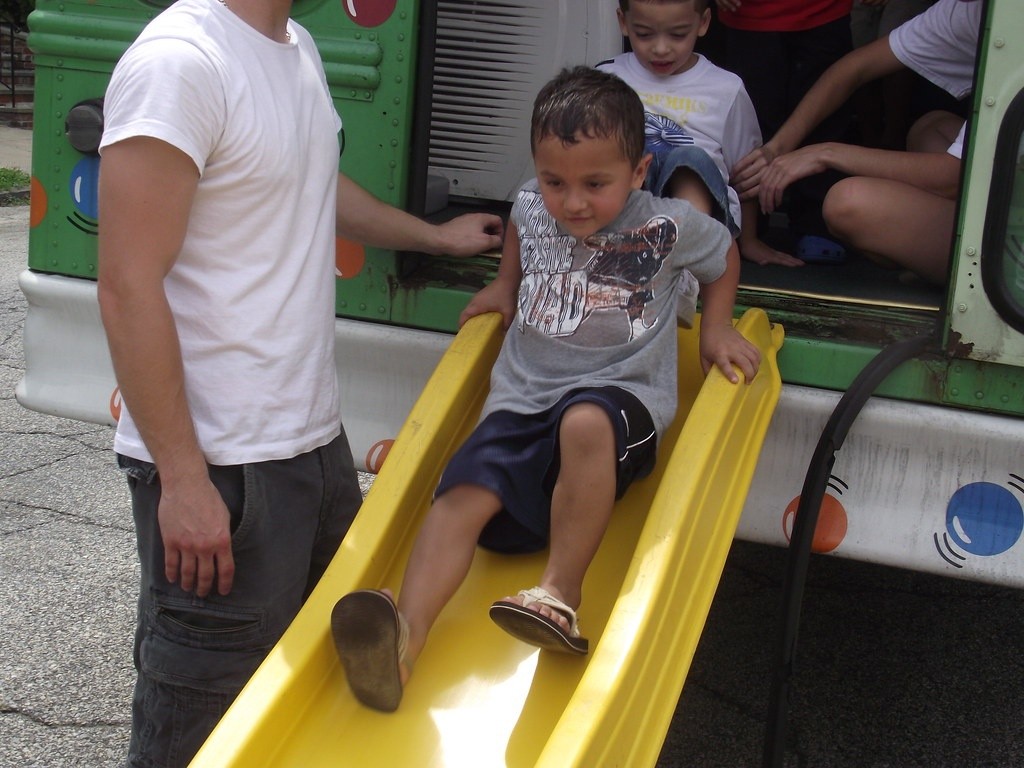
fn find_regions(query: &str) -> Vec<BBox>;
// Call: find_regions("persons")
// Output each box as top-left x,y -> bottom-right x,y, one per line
330,57 -> 765,719
732,0 -> 1024,306
96,0 -> 507,764
711,0 -> 864,264
590,0 -> 806,324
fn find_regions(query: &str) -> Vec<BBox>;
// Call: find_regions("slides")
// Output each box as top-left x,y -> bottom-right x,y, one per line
182,312 -> 788,768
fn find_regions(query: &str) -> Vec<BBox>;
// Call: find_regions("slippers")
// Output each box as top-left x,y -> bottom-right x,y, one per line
794,231 -> 851,264
329,588 -> 411,713
489,585 -> 590,655
